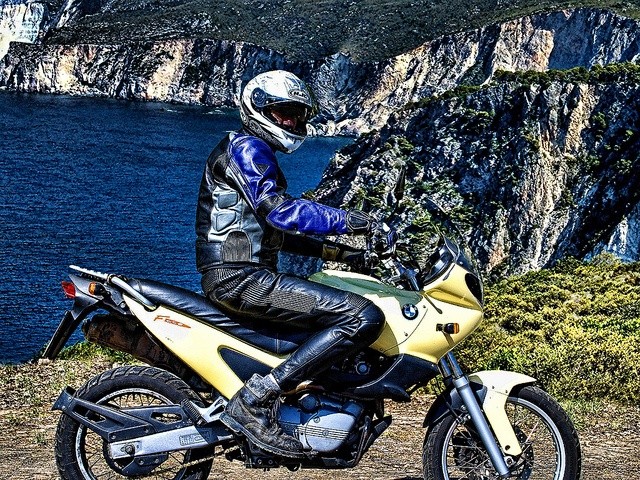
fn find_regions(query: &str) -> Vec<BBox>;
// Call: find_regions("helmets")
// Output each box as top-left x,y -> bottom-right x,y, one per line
236,71 -> 313,153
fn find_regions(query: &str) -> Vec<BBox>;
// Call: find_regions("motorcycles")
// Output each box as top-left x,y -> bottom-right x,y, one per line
40,164 -> 580,474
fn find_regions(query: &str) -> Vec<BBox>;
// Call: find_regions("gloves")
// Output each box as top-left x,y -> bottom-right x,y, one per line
320,240 -> 378,269
345,208 -> 396,252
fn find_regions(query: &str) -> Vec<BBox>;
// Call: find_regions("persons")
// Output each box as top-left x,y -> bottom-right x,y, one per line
195,69 -> 398,458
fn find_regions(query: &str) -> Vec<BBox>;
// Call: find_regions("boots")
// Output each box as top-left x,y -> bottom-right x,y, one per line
221,372 -> 302,458
